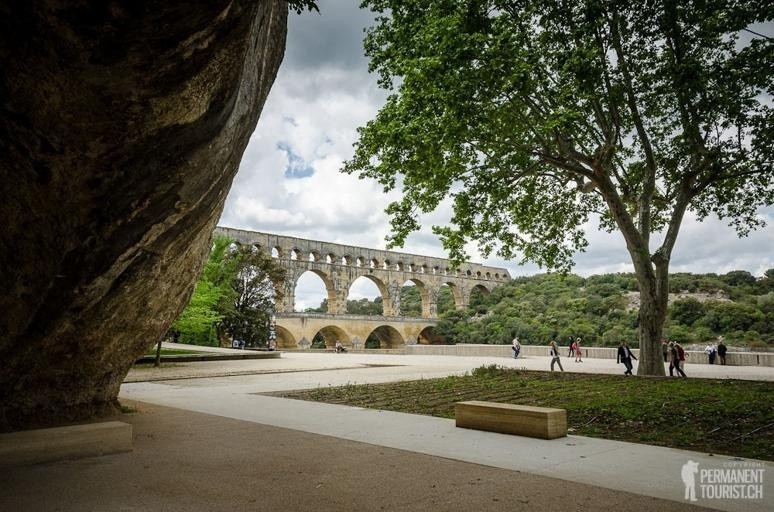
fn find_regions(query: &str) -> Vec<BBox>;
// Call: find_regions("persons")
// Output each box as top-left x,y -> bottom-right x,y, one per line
705,341 -> 727,365
336,339 -> 341,353
662,339 -> 688,377
567,336 -> 583,362
617,339 -> 638,375
512,335 -> 521,359
549,341 -> 564,372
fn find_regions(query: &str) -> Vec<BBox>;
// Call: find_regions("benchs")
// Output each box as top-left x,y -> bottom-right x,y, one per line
0,418 -> 134,470
452,394 -> 570,441
